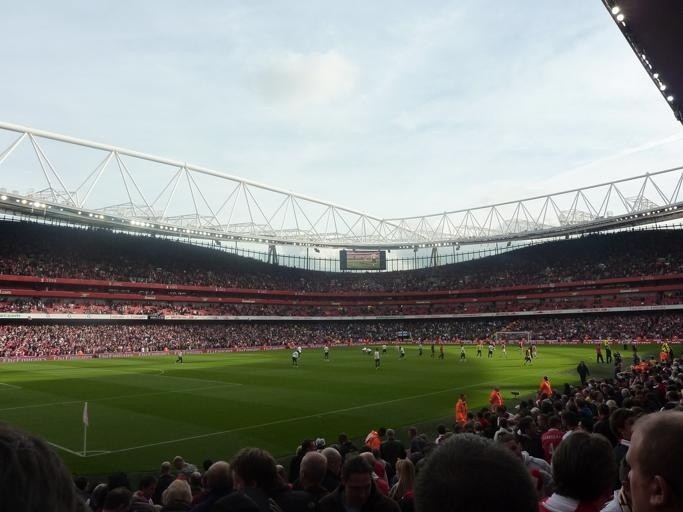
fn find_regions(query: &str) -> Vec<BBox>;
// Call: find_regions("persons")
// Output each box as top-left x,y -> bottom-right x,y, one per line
450,367 -> 681,424
290,349 -> 300,369
577,360 -> 589,384
1,216 -> 683,365
370,349 -> 382,371
1,425 -> 682,511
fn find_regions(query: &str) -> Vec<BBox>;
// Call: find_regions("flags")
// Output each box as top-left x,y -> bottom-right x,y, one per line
81,401 -> 91,427
